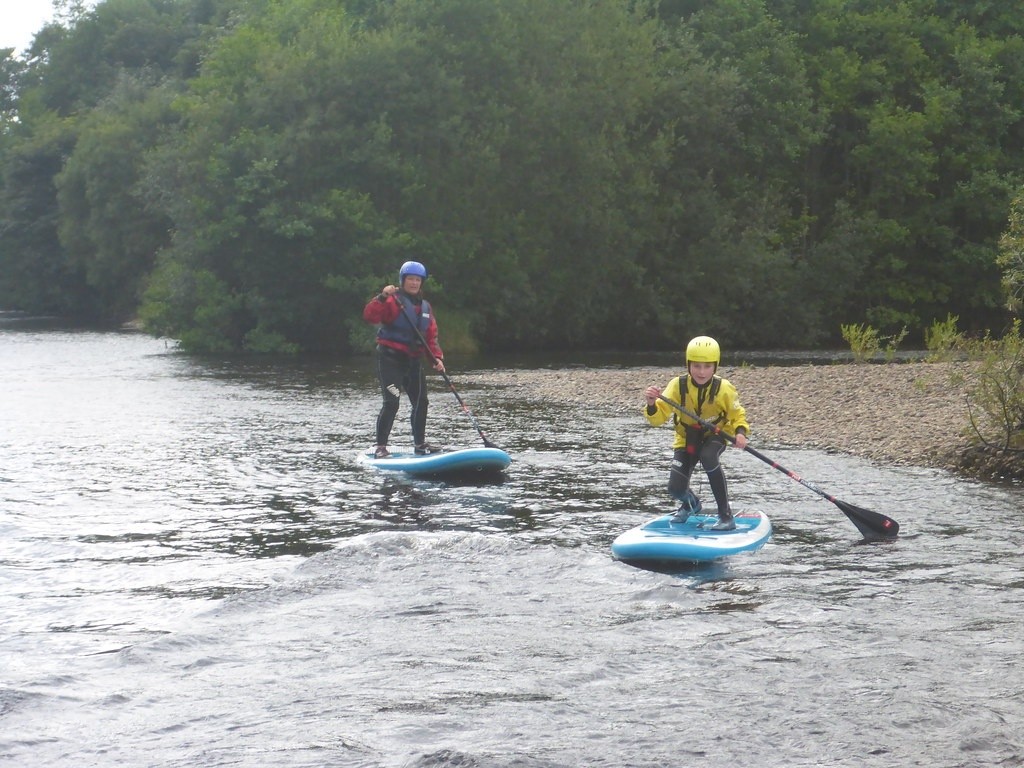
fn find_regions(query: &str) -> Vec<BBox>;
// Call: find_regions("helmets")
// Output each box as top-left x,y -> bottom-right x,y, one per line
685,336 -> 720,374
399,262 -> 426,283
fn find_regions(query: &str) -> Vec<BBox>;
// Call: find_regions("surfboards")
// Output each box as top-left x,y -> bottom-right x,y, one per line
609,507 -> 772,564
356,443 -> 511,477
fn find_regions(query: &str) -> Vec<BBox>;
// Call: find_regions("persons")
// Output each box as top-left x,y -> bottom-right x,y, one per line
644,336 -> 750,531
363,261 -> 445,459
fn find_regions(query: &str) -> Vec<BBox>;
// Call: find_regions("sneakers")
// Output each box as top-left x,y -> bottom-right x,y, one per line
670,499 -> 702,523
712,518 -> 736,531
374,448 -> 393,459
414,443 -> 442,456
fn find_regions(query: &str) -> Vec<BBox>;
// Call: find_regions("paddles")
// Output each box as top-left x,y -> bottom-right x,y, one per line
386,287 -> 500,449
657,394 -> 901,538
